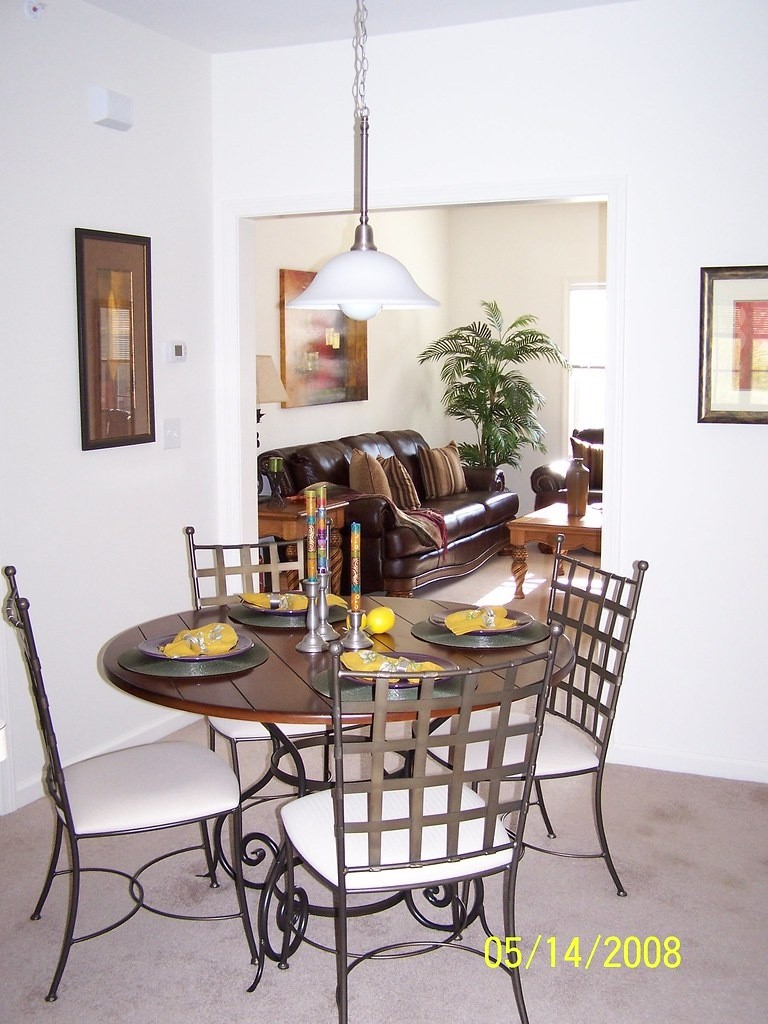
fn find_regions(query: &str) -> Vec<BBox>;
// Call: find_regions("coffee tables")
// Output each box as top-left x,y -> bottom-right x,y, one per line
506,503 -> 604,597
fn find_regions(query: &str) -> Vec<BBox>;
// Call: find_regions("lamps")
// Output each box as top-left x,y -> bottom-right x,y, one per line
285,0 -> 441,320
255,354 -> 289,502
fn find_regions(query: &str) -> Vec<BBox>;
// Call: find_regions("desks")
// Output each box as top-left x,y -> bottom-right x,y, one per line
105,598 -> 577,994
259,496 -> 350,595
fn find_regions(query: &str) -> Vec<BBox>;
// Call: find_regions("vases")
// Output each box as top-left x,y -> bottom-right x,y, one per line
567,457 -> 590,517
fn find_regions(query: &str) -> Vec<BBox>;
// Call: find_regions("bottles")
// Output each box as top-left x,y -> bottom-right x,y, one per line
566,458 -> 590,516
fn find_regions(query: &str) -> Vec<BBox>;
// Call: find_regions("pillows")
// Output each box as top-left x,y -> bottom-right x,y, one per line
570,437 -> 603,488
349,448 -> 393,501
376,455 -> 421,511
417,440 -> 469,500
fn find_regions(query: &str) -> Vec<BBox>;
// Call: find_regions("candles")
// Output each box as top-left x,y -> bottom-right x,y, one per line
269,457 -> 283,473
305,491 -> 318,582
315,486 -> 328,574
350,523 -> 360,612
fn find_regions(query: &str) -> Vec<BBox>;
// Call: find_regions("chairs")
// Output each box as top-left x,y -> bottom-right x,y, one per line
186,517 -> 372,879
272,623 -> 562,1023
413,534 -> 648,941
3,566 -> 262,1003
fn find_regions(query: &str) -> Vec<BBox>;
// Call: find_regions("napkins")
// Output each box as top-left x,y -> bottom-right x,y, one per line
445,606 -> 519,635
238,592 -> 349,609
341,650 -> 445,683
159,622 -> 238,659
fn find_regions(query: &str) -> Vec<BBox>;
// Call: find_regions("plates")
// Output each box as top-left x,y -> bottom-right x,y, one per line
241,600 -> 308,615
137,633 -> 255,661
428,606 -> 535,634
339,652 -> 458,688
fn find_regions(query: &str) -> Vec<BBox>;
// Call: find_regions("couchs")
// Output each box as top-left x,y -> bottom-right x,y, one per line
531,426 -> 604,556
257,430 -> 520,598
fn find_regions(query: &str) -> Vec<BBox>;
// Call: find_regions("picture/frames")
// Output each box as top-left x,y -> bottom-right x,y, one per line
74,229 -> 155,451
698,265 -> 768,426
